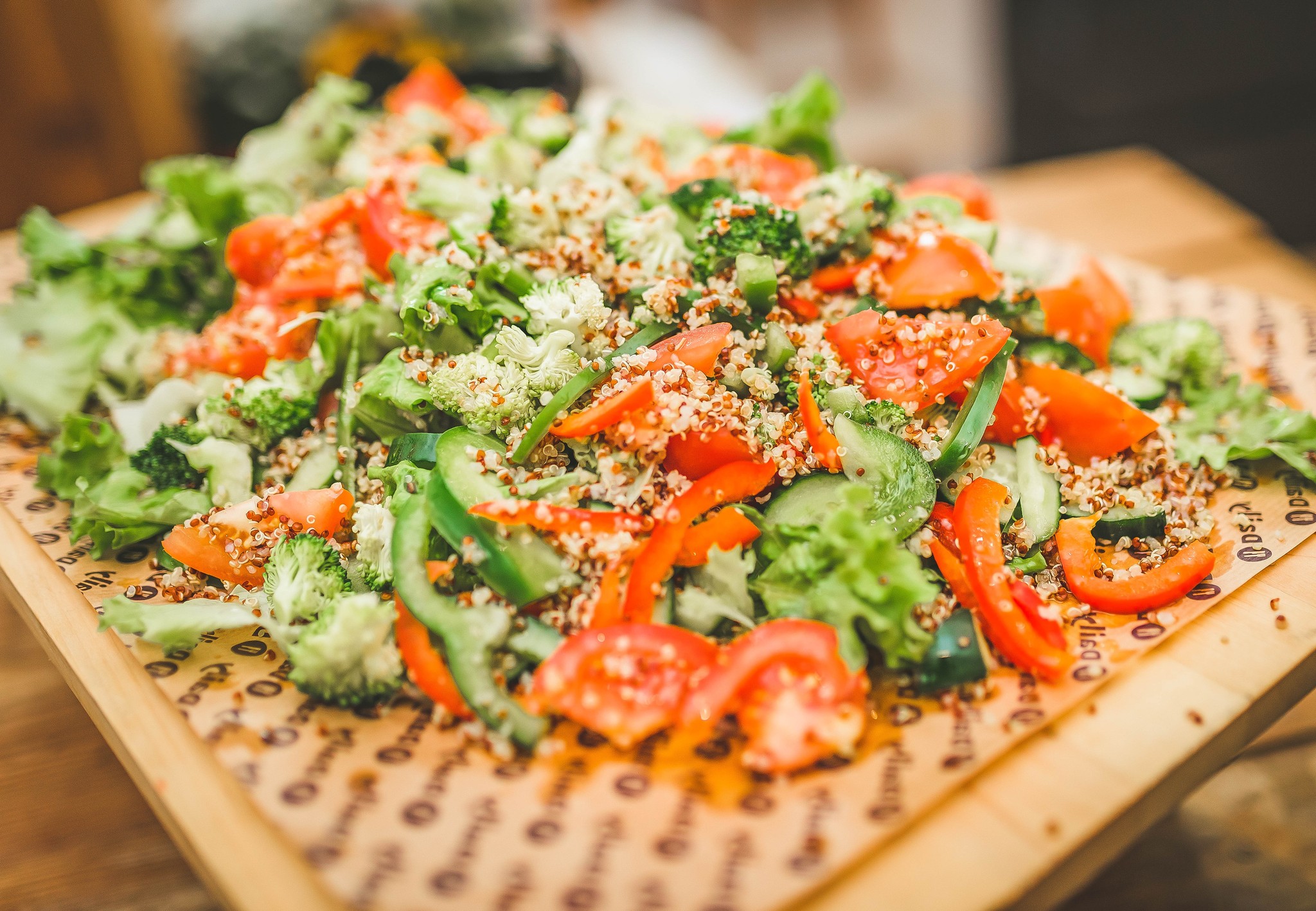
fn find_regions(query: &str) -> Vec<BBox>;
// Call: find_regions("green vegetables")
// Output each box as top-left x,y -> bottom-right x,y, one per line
0,69 -> 1315,711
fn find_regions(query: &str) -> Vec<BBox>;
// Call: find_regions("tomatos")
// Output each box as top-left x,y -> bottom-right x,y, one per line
152,53 -> 1217,759
